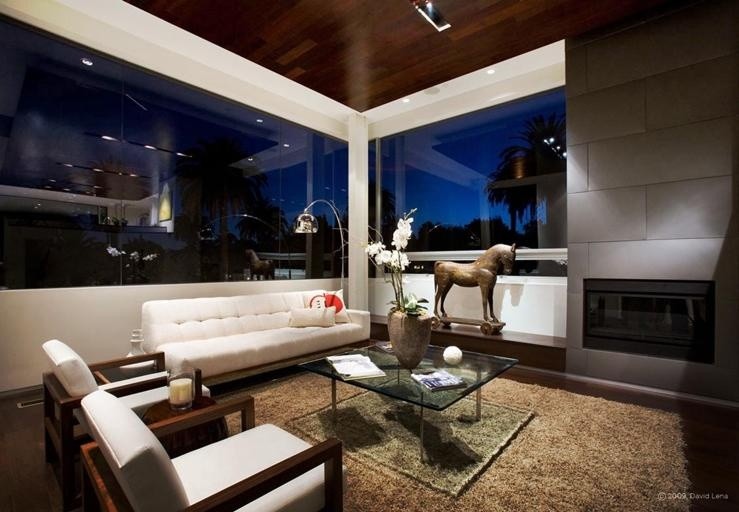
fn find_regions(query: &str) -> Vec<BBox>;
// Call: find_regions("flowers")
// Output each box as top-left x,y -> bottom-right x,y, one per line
106,244 -> 157,272
336,208 -> 429,313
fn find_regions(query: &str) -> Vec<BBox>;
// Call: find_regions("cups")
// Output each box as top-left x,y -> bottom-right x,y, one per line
167,366 -> 194,412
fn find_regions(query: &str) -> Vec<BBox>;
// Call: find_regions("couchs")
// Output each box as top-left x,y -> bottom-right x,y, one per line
140,289 -> 370,381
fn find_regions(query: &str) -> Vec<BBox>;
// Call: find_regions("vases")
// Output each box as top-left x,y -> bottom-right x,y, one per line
127,273 -> 150,285
387,305 -> 432,371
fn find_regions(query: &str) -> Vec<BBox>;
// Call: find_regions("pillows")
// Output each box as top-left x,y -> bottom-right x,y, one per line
288,289 -> 351,328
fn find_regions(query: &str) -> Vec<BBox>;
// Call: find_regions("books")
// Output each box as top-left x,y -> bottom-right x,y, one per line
410,368 -> 468,392
325,353 -> 386,381
376,340 -> 394,353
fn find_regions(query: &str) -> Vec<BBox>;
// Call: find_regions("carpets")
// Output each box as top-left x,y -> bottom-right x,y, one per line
212,345 -> 690,512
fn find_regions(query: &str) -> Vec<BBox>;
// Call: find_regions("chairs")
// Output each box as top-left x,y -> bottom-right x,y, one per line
40,335 -> 350,512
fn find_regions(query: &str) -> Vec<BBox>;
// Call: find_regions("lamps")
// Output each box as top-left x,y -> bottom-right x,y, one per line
197,214 -> 291,279
293,199 -> 344,289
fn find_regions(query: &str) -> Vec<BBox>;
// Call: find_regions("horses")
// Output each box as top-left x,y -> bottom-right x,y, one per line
433,243 -> 516,322
